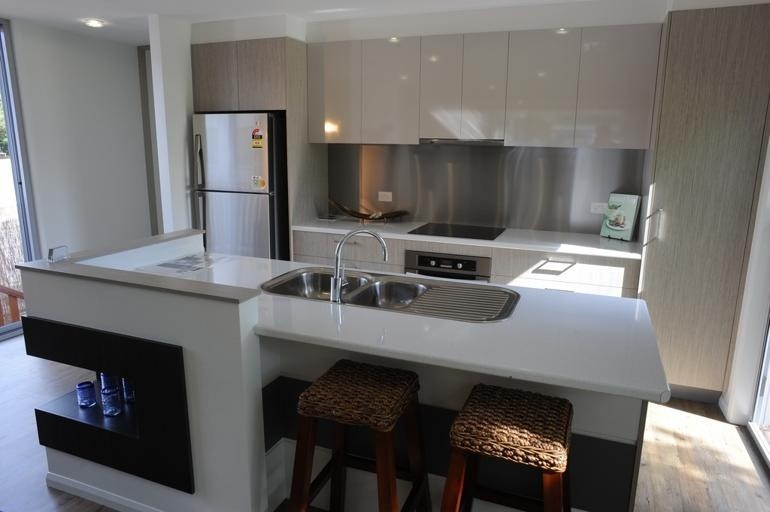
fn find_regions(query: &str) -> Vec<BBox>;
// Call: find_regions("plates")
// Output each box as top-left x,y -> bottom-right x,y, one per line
606,218 -> 630,230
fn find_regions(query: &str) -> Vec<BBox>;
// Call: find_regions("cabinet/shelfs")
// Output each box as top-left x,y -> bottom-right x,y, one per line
505,22 -> 664,150
421,32 -> 508,140
306,35 -> 418,143
190,38 -> 307,111
292,228 -> 407,279
491,245 -> 640,299
635,2 -> 770,393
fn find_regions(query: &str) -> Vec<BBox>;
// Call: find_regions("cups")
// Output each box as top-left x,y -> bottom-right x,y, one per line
100,384 -> 122,416
122,377 -> 136,401
100,371 -> 121,390
76,380 -> 97,407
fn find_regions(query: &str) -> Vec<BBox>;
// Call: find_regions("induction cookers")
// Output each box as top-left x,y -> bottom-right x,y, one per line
408,222 -> 505,240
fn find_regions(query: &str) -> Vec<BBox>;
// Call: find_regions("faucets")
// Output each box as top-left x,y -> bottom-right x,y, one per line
326,230 -> 389,302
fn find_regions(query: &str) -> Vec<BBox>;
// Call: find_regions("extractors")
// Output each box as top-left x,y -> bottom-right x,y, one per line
419,138 -> 504,148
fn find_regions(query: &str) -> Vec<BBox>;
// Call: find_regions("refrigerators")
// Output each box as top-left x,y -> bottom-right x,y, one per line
191,113 -> 284,260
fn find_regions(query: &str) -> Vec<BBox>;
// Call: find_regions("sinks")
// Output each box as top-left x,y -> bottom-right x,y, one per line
345,279 -> 428,310
263,266 -> 370,301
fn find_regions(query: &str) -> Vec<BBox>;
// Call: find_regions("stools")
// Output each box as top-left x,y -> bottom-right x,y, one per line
441,382 -> 574,511
289,358 -> 431,511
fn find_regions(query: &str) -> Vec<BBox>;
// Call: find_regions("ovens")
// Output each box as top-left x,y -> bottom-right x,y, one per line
404,250 -> 492,284
